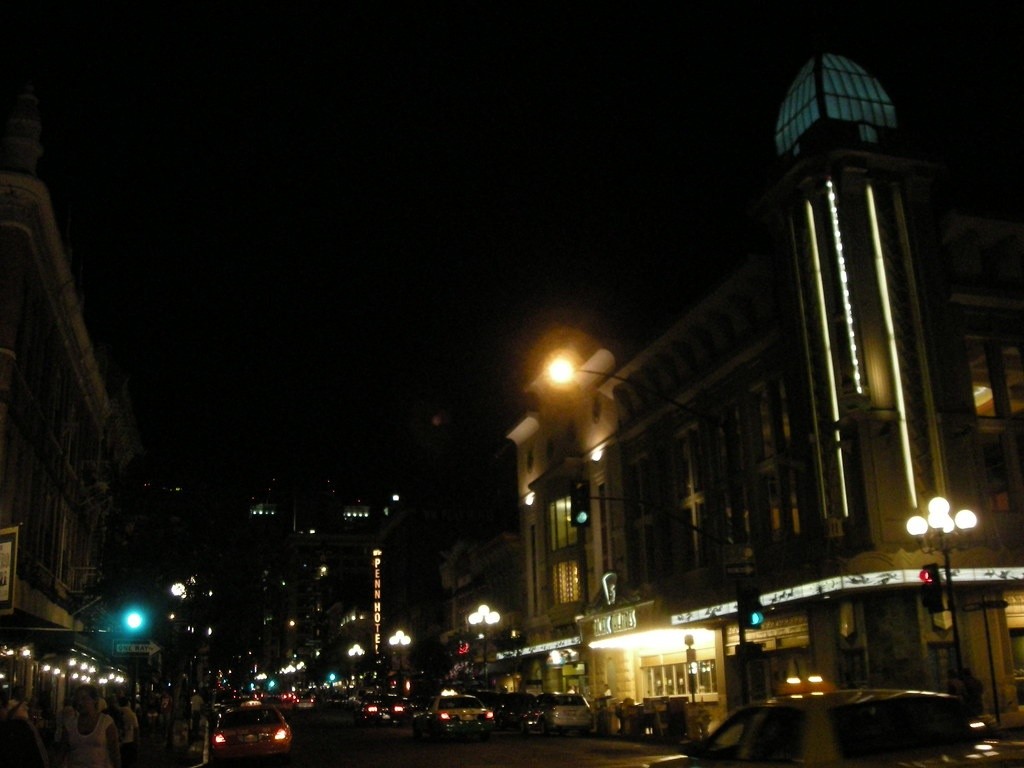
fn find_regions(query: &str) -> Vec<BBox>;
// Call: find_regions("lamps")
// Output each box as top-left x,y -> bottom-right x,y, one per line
820,516 -> 847,539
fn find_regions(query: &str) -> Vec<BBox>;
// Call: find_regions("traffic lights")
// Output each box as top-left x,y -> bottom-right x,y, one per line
920,563 -> 941,587
572,481 -> 591,526
741,587 -> 763,629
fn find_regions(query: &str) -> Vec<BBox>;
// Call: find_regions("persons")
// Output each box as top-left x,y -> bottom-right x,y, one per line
0,684 -> 204,768
603,684 -> 611,697
567,685 -> 576,694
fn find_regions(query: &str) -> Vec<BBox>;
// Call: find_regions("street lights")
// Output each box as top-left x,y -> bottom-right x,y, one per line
388,630 -> 411,692
467,604 -> 501,690
906,495 -> 979,698
348,644 -> 366,689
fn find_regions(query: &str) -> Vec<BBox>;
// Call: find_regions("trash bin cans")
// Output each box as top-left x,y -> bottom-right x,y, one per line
596,696 -> 621,737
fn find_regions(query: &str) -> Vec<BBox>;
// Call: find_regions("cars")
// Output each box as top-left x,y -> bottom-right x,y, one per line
477,691 -> 596,736
210,698 -> 292,759
253,689 -> 408,728
649,690 -> 1024,768
412,695 -> 495,742
